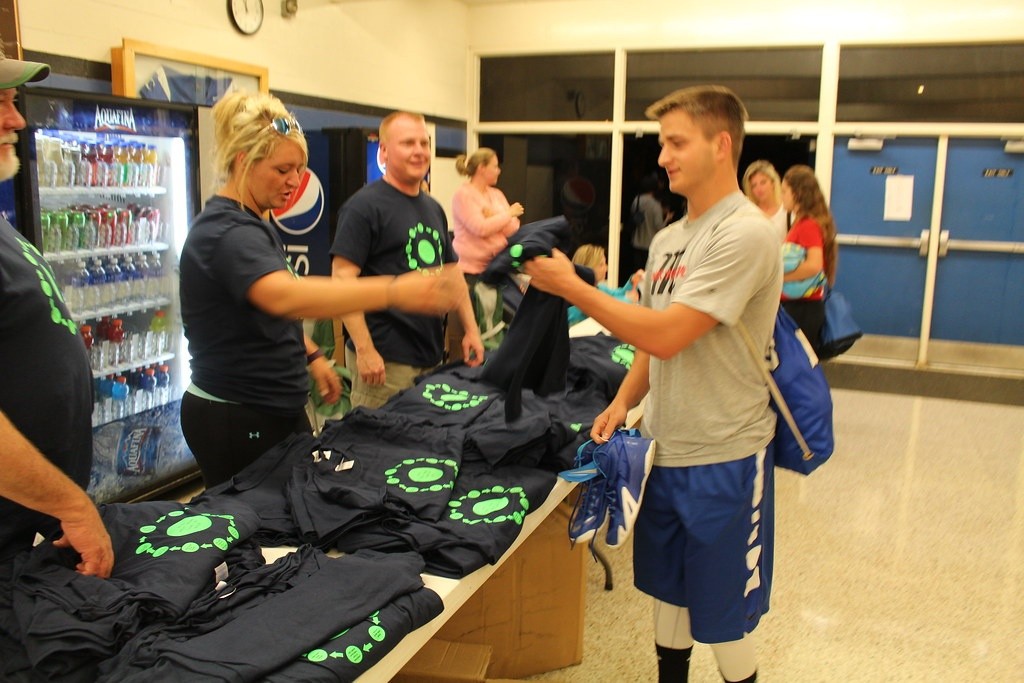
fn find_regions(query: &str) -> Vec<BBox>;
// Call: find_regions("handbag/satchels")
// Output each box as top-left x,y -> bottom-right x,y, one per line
821,289 -> 864,360
631,195 -> 644,226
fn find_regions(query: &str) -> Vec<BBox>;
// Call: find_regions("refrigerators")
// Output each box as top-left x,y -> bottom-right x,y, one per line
19,81 -> 206,505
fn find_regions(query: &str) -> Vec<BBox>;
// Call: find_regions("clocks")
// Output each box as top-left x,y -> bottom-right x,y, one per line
228,0 -> 265,34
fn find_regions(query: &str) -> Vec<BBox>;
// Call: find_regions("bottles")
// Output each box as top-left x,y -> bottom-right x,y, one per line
76,305 -> 170,370
34,131 -> 158,190
92,360 -> 171,427
88,423 -> 195,480
39,191 -> 163,252
46,249 -> 164,314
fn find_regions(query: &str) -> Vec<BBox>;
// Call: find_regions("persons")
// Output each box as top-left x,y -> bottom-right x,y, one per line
572,245 -> 607,286
743,160 -> 796,245
331,111 -> 484,409
780,164 -> 837,348
445,148 -> 524,361
518,87 -> 784,683
179,93 -> 467,488
629,167 -> 675,249
0,51 -> 114,578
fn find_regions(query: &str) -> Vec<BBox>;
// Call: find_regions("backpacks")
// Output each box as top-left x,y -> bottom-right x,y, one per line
654,225 -> 834,476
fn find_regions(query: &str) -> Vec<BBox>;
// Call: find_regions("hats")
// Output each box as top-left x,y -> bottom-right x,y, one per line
0,51 -> 51,89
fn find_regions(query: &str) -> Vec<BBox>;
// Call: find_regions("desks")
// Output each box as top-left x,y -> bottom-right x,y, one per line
261,473 -> 592,683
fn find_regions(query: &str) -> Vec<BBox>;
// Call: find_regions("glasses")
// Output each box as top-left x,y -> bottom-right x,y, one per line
257,117 -> 300,135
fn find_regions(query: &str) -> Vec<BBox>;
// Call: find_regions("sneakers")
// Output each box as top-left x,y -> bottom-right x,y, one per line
594,435 -> 656,549
568,429 -> 641,563
